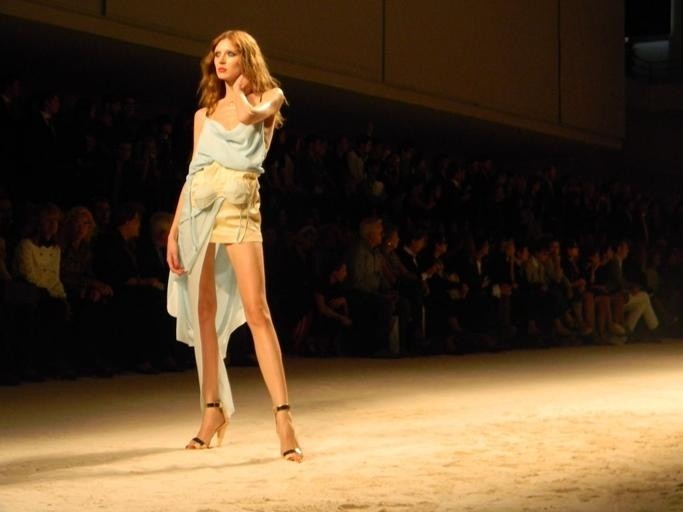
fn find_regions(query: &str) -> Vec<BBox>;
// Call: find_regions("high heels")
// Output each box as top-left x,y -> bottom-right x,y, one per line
273,404 -> 305,465
183,399 -> 229,450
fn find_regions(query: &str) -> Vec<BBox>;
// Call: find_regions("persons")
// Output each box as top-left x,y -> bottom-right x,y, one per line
165,29 -> 302,461
0,81 -> 304,386
302,135 -> 683,357
